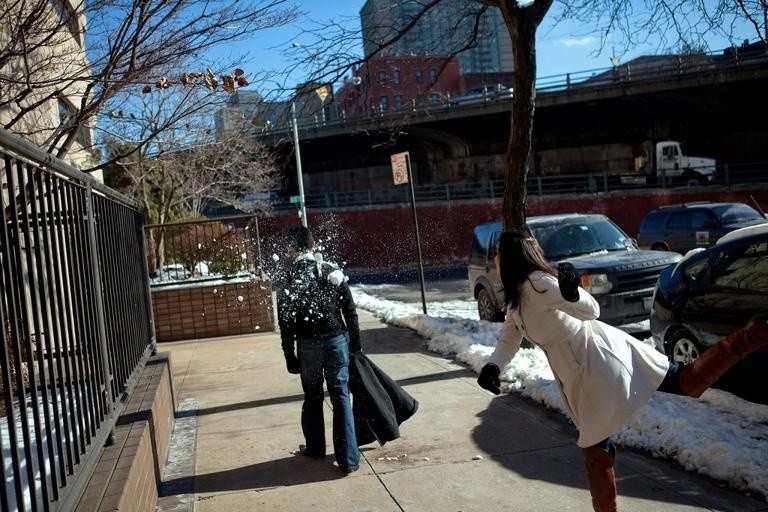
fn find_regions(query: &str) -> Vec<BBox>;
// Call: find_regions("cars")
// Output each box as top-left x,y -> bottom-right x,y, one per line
448,83 -> 514,108
637,199 -> 767,254
649,222 -> 768,404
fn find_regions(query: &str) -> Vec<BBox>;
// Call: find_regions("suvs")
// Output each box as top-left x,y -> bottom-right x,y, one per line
469,212 -> 686,332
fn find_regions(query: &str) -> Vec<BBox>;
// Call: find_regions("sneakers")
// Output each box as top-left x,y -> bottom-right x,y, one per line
299,444 -> 326,459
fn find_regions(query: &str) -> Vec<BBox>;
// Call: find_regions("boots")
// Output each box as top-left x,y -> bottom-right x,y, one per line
583,442 -> 617,512
680,315 -> 767,399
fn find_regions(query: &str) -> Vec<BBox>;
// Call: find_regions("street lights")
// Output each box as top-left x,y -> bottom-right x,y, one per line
292,76 -> 363,226
293,42 -> 326,127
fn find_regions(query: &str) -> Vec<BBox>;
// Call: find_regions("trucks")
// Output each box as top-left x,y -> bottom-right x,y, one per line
441,140 -> 720,200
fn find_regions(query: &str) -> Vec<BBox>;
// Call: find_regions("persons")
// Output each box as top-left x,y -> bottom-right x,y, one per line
274,223 -> 361,474
477,230 -> 768,512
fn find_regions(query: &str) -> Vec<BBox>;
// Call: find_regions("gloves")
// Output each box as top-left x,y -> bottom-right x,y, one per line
286,357 -> 299,373
558,262 -> 580,302
478,363 -> 500,395
